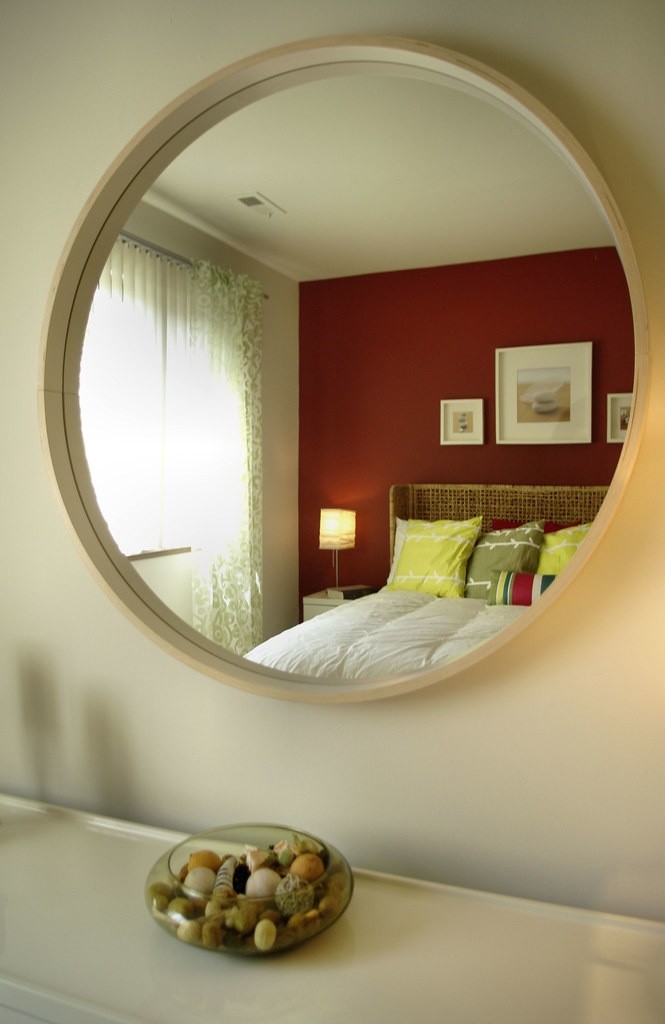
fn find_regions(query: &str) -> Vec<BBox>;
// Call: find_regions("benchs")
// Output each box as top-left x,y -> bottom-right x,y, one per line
489,570 -> 556,604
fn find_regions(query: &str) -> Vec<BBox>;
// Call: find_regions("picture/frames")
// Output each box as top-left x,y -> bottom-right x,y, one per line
606,392 -> 633,444
439,398 -> 484,445
495,340 -> 592,444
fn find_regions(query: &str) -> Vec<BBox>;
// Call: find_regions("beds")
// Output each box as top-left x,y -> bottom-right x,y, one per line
241,484 -> 609,679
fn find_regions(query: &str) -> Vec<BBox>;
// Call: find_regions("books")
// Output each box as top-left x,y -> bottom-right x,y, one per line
327,585 -> 371,599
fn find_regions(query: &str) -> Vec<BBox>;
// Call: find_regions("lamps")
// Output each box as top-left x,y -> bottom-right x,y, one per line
319,508 -> 356,589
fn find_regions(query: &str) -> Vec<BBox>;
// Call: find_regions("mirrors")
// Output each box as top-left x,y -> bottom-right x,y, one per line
44,34 -> 654,704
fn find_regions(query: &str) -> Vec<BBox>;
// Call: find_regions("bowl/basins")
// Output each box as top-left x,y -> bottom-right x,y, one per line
533,392 -> 558,413
144,822 -> 354,956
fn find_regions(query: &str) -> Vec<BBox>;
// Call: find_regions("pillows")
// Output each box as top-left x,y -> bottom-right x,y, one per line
392,515 -> 482,598
537,522 -> 593,575
386,516 -> 408,585
464,519 -> 545,599
